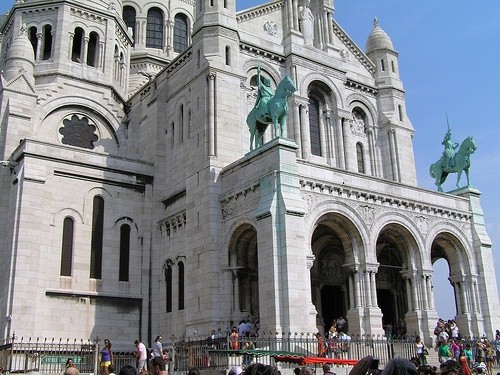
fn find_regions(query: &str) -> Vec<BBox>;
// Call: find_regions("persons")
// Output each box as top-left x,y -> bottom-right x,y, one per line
314,316 -> 352,358
99,338 -> 113,375
434,318 -> 500,375
441,129 -> 458,167
382,335 -> 437,375
207,313 -> 260,350
130,336 -> 170,373
382,317 -> 407,340
108,357 -> 170,375
185,346 -> 211,375
229,363 -> 336,375
257,64 -> 274,104
65,358 -> 74,368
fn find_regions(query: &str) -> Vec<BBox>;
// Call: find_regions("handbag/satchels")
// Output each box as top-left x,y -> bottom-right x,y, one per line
421,342 -> 429,355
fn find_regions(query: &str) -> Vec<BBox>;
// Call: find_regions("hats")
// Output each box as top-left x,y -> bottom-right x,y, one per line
466,344 -> 471,347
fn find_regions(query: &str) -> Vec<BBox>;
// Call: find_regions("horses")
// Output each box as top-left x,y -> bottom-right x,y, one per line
429,136 -> 477,193
245,74 -> 297,152
